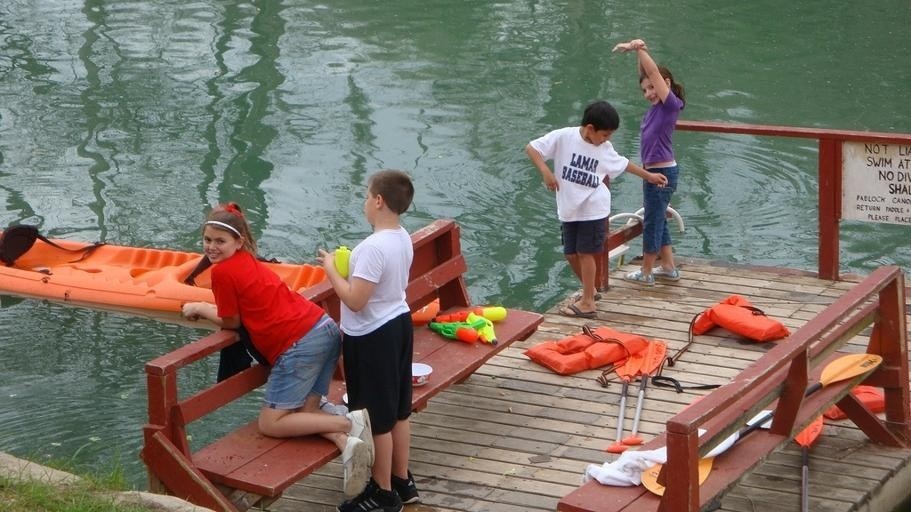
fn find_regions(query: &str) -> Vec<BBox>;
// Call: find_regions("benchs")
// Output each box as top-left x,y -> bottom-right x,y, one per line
126,216 -> 545,512
549,262 -> 909,511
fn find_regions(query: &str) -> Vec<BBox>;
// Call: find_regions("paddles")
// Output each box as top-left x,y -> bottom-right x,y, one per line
622,339 -> 667,445
640,353 -> 883,496
608,353 -> 640,453
794,414 -> 823,511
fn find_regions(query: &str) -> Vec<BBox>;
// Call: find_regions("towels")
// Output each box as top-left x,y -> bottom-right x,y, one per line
570,425 -> 743,489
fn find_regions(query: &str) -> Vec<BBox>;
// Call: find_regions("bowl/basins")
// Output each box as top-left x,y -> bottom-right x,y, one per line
411,361 -> 434,388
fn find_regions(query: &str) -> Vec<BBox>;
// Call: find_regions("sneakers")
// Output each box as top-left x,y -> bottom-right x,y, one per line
391,469 -> 419,504
652,264 -> 680,281
345,408 -> 375,467
623,267 -> 655,286
335,477 -> 404,512
341,436 -> 369,496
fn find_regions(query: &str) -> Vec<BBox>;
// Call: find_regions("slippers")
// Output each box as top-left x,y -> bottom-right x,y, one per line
574,293 -> 601,302
559,304 -> 597,318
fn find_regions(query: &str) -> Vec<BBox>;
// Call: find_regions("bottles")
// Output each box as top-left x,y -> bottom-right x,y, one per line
335,244 -> 352,279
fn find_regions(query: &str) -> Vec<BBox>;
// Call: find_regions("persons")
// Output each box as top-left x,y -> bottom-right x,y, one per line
181,200 -> 377,498
611,38 -> 686,287
526,101 -> 668,319
315,169 -> 419,512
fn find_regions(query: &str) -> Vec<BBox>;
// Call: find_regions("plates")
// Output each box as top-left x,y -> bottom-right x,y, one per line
342,392 -> 349,405
